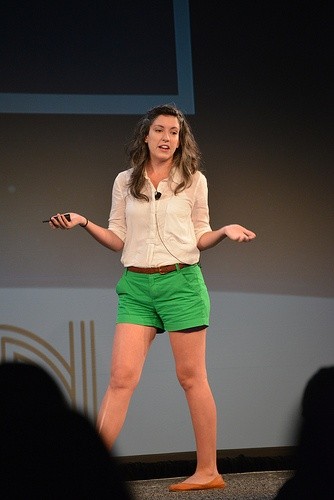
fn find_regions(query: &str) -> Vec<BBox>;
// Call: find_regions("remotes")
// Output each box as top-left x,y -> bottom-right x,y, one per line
42,214 -> 70,224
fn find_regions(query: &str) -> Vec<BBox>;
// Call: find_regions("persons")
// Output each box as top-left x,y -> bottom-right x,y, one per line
273,366 -> 334,500
0,358 -> 133,500
49,105 -> 256,492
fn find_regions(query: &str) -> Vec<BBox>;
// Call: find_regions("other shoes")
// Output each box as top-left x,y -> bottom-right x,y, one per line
169,475 -> 227,491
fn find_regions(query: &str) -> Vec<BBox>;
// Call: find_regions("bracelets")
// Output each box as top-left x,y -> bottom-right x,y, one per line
81,219 -> 88,228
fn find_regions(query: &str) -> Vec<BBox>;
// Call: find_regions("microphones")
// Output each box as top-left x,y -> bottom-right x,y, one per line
155,192 -> 161,200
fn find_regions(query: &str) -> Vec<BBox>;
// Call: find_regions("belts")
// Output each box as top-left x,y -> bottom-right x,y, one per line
126,264 -> 186,275
